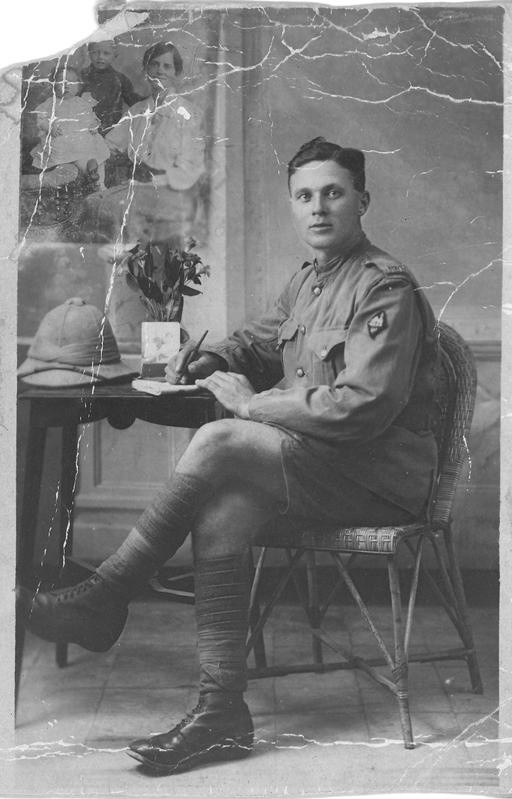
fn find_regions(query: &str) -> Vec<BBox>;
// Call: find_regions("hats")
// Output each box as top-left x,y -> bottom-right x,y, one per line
17,295 -> 140,388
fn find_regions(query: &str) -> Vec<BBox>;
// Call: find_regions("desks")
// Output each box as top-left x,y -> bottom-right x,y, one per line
13,381 -> 232,725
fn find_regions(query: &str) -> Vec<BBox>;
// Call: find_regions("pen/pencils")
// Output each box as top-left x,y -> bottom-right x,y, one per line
174,329 -> 208,385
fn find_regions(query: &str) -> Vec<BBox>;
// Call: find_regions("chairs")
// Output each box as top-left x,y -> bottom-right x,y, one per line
244,320 -> 485,749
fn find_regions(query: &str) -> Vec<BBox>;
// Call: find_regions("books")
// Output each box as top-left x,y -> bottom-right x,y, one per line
131,379 -> 202,398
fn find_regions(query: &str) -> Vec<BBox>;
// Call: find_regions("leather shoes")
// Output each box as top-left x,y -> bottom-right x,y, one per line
126,689 -> 254,776
16,571 -> 129,658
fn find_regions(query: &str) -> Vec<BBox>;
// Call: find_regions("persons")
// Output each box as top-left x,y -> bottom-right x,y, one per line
70,42 -> 209,244
27,67 -> 112,192
76,41 -> 150,136
20,136 -> 439,774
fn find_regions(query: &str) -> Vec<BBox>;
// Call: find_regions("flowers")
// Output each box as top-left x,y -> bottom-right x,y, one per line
129,238 -> 211,321
